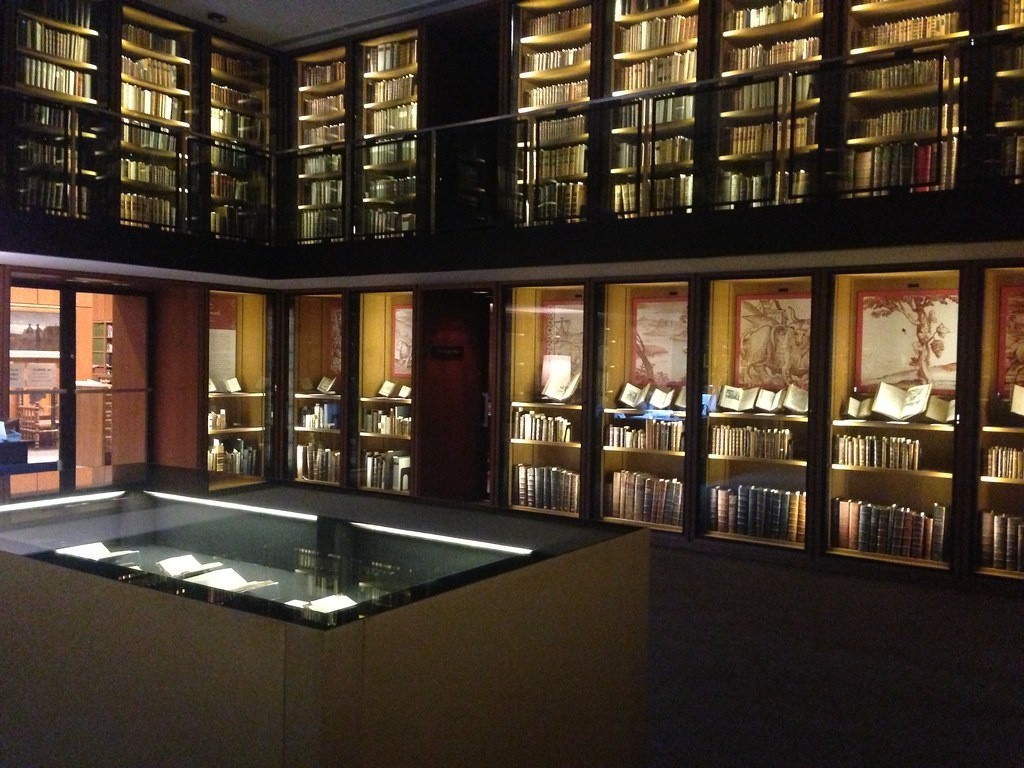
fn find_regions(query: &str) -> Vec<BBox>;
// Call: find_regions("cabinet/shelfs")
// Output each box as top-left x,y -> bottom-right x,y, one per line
509,400 -> 583,515
601,403 -> 687,532
13,0 -> 108,224
710,0 -> 834,215
114,3 -> 194,241
292,390 -> 342,484
358,395 -> 412,492
971,421 -> 1024,571
838,0 -> 968,202
358,24 -> 423,240
508,0 -> 599,235
75,385 -> 110,467
829,414 -> 955,568
703,411 -> 808,551
291,45 -> 354,249
985,0 -> 1024,194
92,320 -> 113,383
205,32 -> 278,251
602,0 -> 704,223
208,385 -> 264,487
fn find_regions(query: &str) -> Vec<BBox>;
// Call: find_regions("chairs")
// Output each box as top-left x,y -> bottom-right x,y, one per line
16,387 -> 61,448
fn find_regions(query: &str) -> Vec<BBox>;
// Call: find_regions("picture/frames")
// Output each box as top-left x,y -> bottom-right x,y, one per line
728,279 -> 813,395
623,285 -> 687,390
985,269 -> 1024,399
848,273 -> 960,404
384,292 -> 412,397
533,288 -> 583,405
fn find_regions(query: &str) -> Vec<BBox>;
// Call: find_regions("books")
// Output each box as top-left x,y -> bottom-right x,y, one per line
119,21 -> 185,232
206,378 -> 263,476
293,376 -> 344,484
708,374 -> 1024,570
612,0 -> 699,219
304,593 -> 357,613
992,0 -> 1024,186
156,554 -> 279,600
842,0 -> 965,198
298,60 -> 345,239
55,542 -> 139,564
209,54 -> 268,240
516,4 -> 593,227
11,0 -> 97,220
713,0 -> 823,210
358,380 -> 413,494
511,373 -> 582,513
361,39 -> 417,235
602,382 -> 688,527
